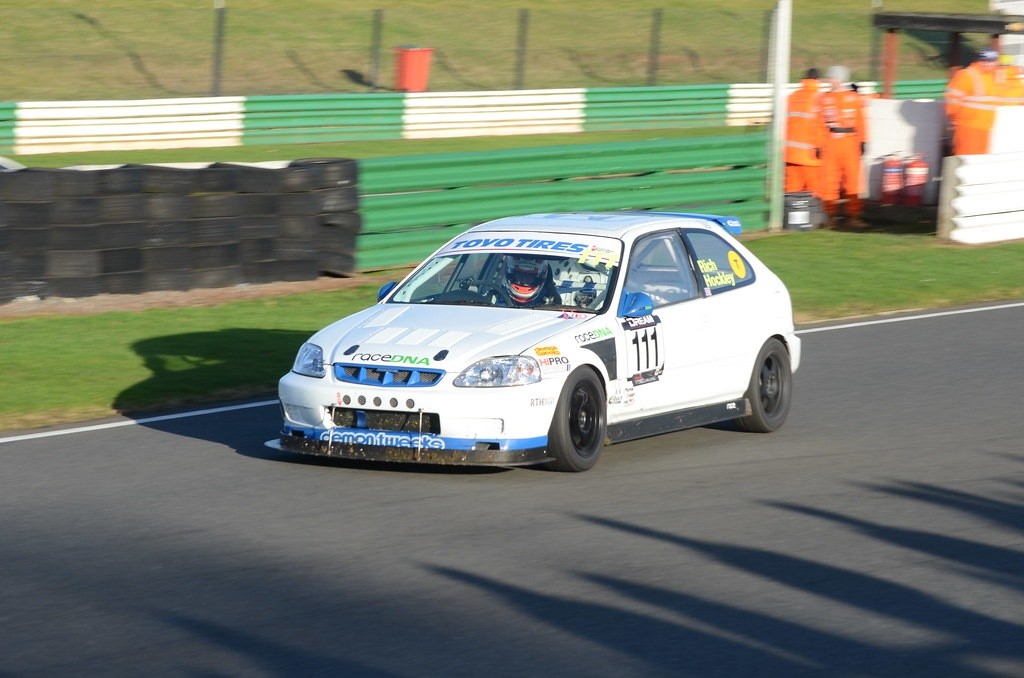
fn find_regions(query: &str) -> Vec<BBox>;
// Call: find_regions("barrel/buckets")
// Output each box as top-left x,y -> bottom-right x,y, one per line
395,46 -> 436,92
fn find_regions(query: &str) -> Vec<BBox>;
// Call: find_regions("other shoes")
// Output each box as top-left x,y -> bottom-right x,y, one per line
844,219 -> 872,229
821,218 -> 840,229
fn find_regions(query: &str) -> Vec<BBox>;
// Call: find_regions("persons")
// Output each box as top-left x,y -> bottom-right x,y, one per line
782,67 -> 827,203
819,66 -> 869,230
944,50 -> 1023,156
459,253 -> 555,306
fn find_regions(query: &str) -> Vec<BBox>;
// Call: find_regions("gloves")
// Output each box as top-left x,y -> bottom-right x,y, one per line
459,277 -> 479,294
816,148 -> 823,160
860,142 -> 867,156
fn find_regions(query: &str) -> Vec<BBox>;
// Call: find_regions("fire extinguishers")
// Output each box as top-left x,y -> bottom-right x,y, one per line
903,152 -> 929,206
876,148 -> 906,205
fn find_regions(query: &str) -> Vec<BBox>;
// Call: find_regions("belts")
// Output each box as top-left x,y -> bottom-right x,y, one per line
830,128 -> 855,133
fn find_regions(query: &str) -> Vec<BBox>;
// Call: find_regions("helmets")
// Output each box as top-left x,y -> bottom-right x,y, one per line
973,49 -> 1000,62
827,66 -> 848,80
502,253 -> 549,305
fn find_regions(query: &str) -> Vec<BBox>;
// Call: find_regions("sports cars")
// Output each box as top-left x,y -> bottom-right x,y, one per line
261,209 -> 800,476
